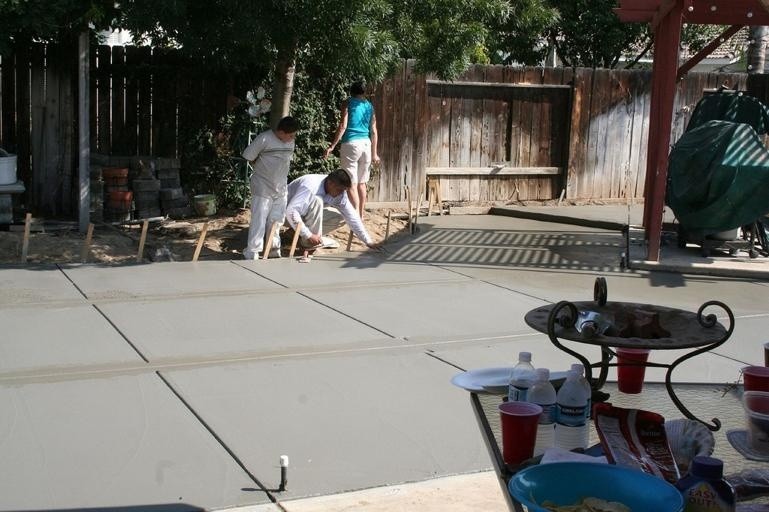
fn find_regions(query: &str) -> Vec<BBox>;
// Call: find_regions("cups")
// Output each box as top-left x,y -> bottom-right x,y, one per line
743,366 -> 769,411
764,342 -> 769,366
496,400 -> 543,463
616,345 -> 648,393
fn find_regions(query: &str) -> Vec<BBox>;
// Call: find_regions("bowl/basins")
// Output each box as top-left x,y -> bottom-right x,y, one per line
506,463 -> 685,512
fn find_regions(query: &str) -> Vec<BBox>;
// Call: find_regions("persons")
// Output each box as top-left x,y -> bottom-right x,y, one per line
323,82 -> 382,221
283,169 -> 379,258
241,117 -> 299,259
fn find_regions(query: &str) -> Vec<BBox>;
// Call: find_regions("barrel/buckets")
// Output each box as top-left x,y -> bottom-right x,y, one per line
0,154 -> 17,185
195,194 -> 217,216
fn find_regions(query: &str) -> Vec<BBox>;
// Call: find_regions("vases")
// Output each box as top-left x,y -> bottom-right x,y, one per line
192,190 -> 220,218
101,166 -> 133,212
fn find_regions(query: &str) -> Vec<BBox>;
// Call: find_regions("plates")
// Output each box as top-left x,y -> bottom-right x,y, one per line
580,418 -> 716,478
725,428 -> 767,461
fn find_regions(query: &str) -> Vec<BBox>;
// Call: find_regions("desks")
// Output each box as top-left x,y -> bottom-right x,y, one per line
1,178 -> 26,225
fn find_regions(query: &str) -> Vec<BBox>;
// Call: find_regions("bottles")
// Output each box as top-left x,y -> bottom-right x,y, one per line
509,348 -> 592,452
675,455 -> 736,511
